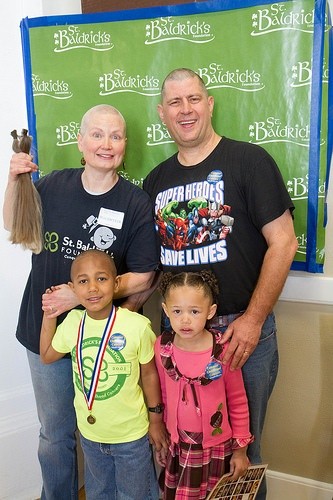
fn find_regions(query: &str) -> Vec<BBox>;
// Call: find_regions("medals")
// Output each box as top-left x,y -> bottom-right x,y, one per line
87,415 -> 95,425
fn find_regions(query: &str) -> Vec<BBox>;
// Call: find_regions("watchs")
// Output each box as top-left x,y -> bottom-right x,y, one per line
148,403 -> 165,414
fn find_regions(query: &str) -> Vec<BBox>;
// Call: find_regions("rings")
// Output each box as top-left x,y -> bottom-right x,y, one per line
245,351 -> 251,355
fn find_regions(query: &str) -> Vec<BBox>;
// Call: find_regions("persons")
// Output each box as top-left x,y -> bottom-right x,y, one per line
3,105 -> 158,500
40,250 -> 171,500
152,269 -> 255,500
121,68 -> 298,500
